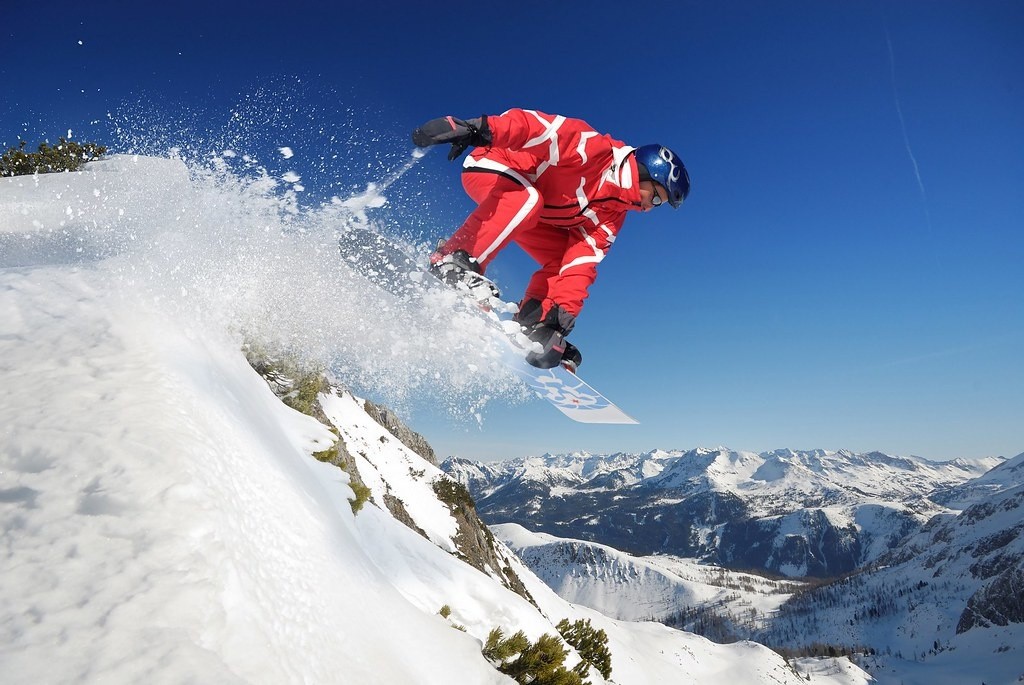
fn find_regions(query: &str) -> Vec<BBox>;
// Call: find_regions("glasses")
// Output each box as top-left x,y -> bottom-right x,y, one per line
650,181 -> 665,208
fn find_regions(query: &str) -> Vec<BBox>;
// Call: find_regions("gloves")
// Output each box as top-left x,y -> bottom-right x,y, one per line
524,306 -> 576,369
413,114 -> 491,161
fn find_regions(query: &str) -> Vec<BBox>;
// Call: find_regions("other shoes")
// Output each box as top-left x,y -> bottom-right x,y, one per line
515,312 -> 590,365
432,251 -> 502,298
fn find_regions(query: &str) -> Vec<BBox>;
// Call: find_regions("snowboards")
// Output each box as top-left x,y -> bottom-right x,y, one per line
338,228 -> 639,426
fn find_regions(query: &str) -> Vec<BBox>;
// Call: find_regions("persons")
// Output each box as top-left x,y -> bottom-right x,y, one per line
411,107 -> 692,384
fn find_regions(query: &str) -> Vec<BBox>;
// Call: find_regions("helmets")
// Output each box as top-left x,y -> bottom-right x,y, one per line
636,142 -> 690,211
